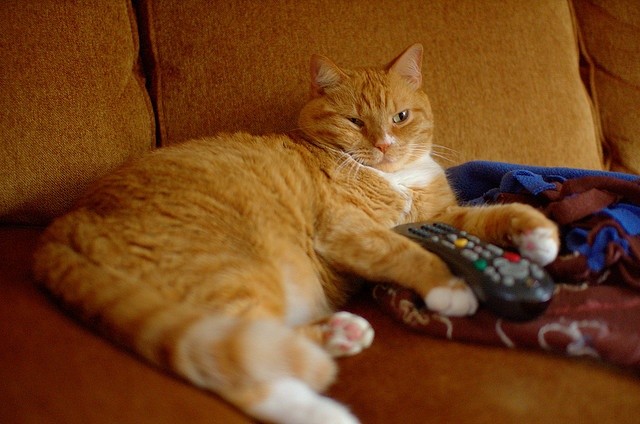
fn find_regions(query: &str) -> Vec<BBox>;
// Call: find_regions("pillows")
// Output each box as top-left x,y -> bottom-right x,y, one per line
572,0 -> 640,174
1,0 -> 156,219
140,0 -> 608,170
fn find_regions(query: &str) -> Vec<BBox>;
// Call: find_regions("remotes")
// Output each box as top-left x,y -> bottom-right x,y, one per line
389,216 -> 556,325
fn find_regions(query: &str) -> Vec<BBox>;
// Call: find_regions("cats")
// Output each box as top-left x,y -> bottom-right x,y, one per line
33,43 -> 560,424
